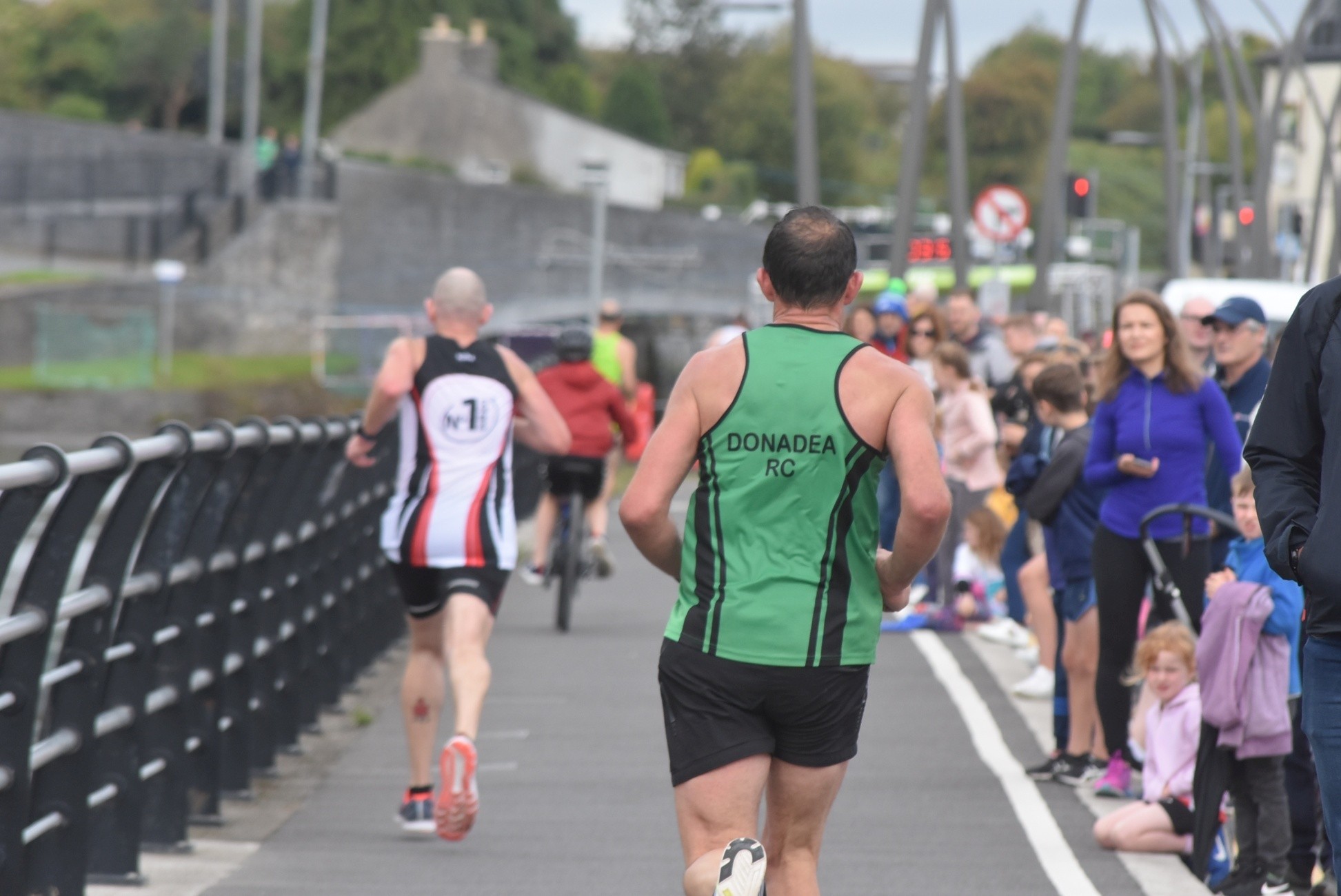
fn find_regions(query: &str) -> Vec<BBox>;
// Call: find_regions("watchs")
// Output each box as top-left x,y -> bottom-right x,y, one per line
347,417 -> 378,441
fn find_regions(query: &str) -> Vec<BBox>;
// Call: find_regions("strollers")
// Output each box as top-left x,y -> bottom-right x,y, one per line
1143,505 -> 1247,633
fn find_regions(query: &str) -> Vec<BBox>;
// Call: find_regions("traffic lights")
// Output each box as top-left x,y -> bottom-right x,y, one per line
1066,173 -> 1091,217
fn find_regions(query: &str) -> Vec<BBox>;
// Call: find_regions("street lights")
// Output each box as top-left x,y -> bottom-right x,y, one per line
579,152 -> 609,335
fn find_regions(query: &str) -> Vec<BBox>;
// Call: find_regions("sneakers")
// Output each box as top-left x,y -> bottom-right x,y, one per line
435,735 -> 480,841
1094,750 -> 1132,799
1056,756 -> 1109,787
1222,865 -> 1294,896
1025,751 -> 1089,781
1207,821 -> 1234,891
399,784 -> 437,835
712,837 -> 767,896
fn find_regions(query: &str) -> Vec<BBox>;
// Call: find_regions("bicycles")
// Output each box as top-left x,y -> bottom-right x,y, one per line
540,434 -> 624,631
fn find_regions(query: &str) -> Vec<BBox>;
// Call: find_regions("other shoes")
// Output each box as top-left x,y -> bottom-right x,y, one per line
589,533 -> 615,578
910,600 -> 943,615
1017,646 -> 1039,665
934,603 -> 962,631
519,563 -> 549,589
1012,665 -> 1056,698
976,616 -> 1031,648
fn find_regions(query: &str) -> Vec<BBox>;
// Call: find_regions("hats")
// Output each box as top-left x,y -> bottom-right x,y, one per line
1202,296 -> 1266,328
873,290 -> 907,321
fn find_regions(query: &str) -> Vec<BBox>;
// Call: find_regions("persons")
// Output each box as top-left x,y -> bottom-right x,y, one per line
343,264 -> 571,845
847,279 -> 1341,895
515,303 -> 639,590
618,205 -> 952,896
